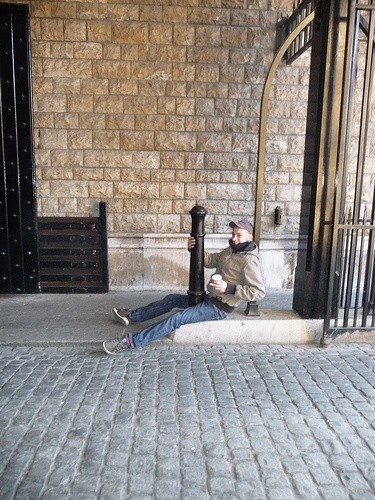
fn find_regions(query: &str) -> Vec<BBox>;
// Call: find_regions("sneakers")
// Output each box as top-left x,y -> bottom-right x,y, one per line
101,332 -> 133,354
112,306 -> 129,326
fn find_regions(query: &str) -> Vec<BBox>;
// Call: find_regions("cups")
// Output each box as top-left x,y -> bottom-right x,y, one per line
211,273 -> 222,283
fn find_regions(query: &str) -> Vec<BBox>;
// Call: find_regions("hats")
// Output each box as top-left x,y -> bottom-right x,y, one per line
228,218 -> 253,234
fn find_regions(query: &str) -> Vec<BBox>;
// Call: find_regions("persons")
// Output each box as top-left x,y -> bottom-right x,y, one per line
102,218 -> 267,354
